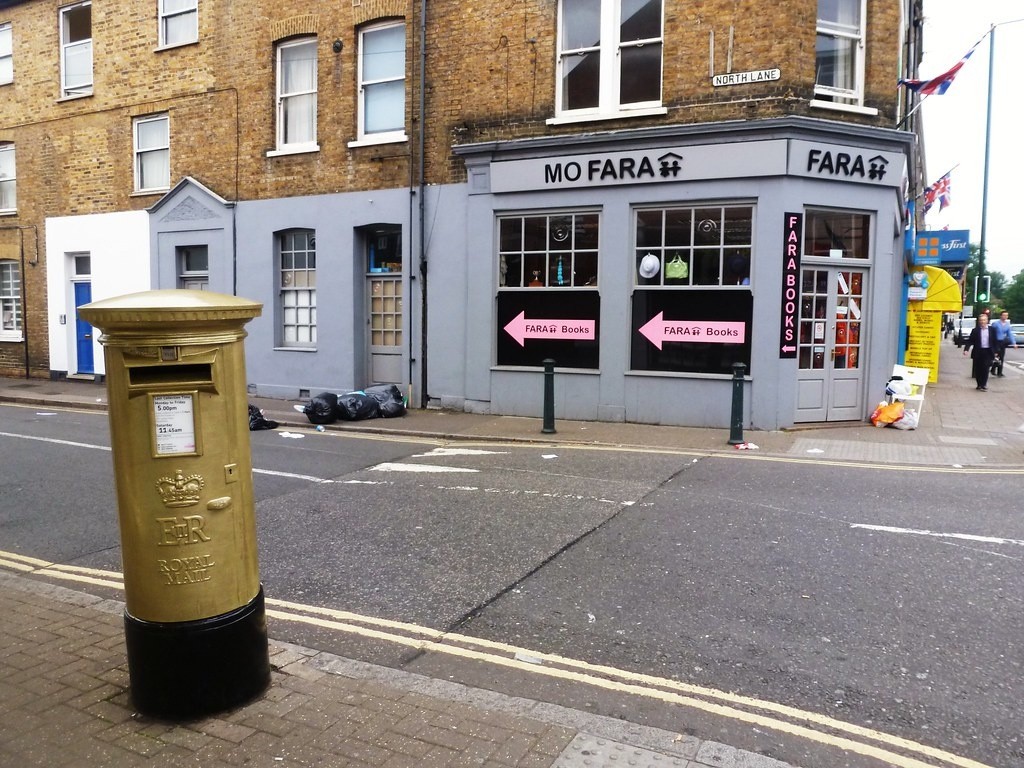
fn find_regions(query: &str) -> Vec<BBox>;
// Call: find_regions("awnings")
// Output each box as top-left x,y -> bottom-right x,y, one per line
907,263 -> 964,314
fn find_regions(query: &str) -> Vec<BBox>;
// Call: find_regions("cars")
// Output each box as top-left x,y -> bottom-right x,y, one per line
953,318 -> 1024,347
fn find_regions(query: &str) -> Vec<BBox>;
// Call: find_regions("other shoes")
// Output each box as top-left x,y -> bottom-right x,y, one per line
995,373 -> 1005,377
990,370 -> 996,376
976,385 -> 988,392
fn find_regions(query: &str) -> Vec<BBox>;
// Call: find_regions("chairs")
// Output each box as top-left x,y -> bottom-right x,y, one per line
888,364 -> 931,428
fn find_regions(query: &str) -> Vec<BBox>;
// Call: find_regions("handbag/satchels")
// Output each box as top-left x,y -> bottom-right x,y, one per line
992,357 -> 1002,367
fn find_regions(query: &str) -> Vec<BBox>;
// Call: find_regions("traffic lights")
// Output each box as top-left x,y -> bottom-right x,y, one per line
973,276 -> 990,303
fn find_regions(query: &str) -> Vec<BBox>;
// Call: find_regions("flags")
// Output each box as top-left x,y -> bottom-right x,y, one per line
923,169 -> 952,214
896,32 -> 990,97
922,186 -> 936,214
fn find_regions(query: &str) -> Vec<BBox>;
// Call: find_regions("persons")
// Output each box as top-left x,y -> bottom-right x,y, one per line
961,313 -> 999,392
990,311 -> 1020,378
